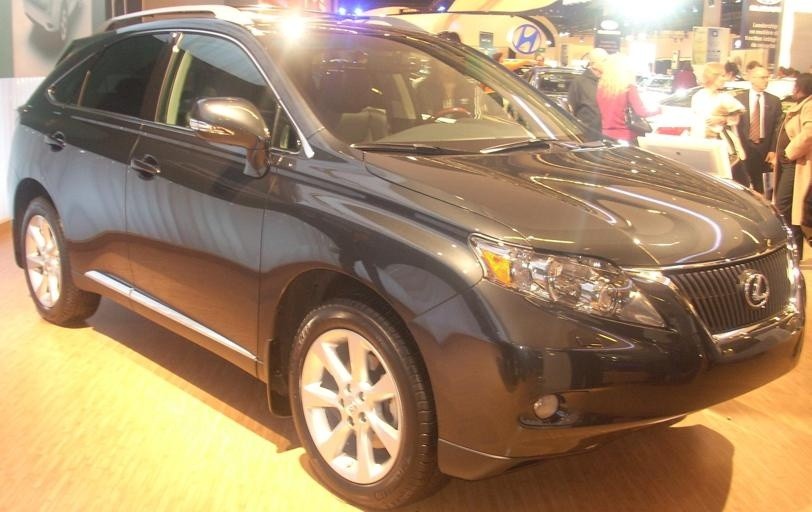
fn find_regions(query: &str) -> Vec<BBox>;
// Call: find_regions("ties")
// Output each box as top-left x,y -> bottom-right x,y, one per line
748,94 -> 763,146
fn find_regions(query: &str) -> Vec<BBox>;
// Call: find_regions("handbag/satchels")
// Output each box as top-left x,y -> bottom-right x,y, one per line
626,87 -> 653,136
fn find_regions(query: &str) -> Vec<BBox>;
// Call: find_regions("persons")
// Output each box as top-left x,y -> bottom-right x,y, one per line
416,30 -> 477,120
480,53 -> 504,116
672,60 -> 696,94
571,47 -> 660,148
690,59 -> 812,266
534,53 -> 546,66
